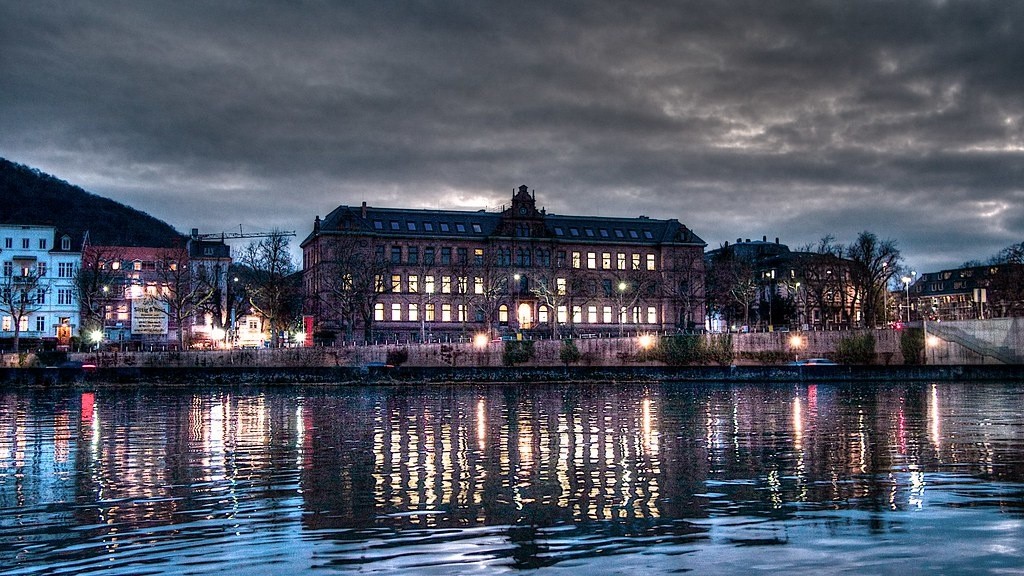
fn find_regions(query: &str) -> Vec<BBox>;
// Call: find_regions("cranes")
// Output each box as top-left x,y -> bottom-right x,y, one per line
187,228 -> 296,253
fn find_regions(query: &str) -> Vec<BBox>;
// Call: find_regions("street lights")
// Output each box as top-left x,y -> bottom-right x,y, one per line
232,277 -> 239,348
795,282 -> 801,331
513,274 -> 521,337
93,331 -> 101,367
901,277 -> 912,322
102,286 -> 110,349
618,282 -> 625,337
